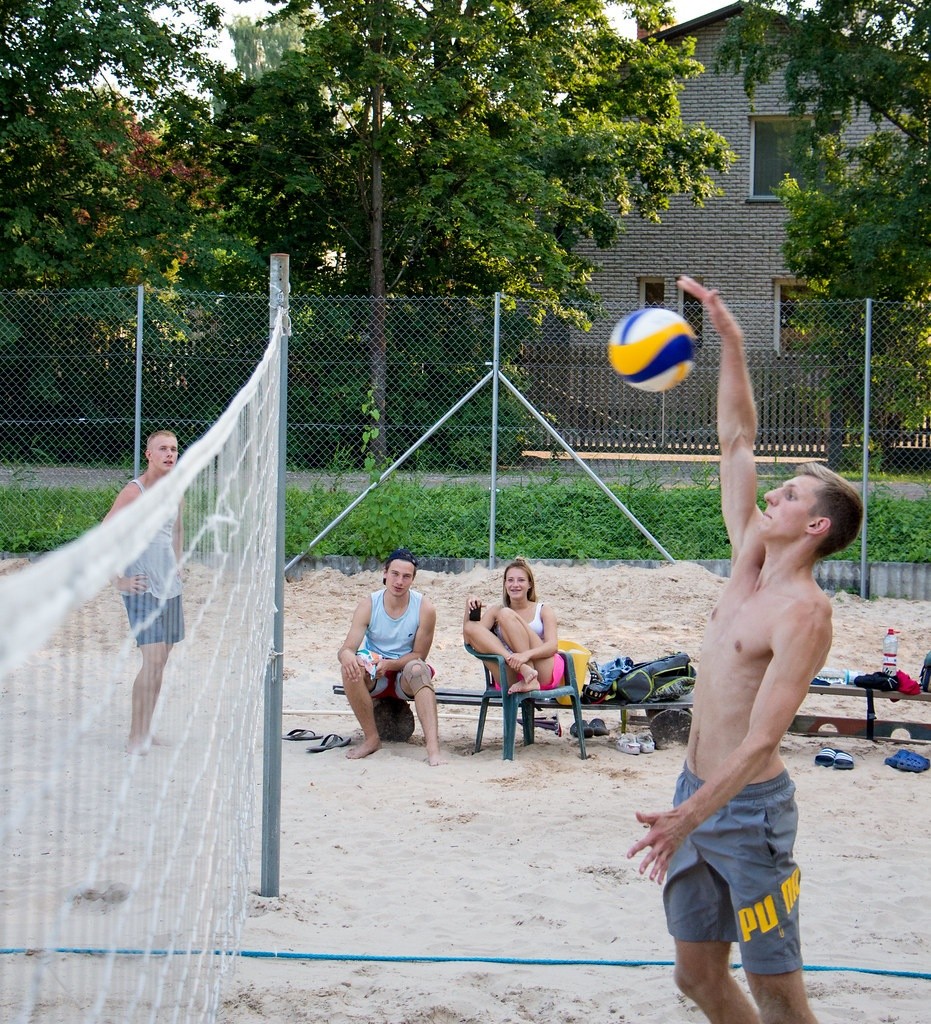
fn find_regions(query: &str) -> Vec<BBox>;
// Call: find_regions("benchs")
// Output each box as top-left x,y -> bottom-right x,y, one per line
807,686 -> 931,738
329,686 -> 692,742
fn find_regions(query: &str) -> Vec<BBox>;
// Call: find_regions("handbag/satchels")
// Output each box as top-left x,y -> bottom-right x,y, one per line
854,672 -> 899,692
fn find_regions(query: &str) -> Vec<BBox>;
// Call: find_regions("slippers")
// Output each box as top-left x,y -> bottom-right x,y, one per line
282,729 -> 322,741
607,733 -> 640,754
834,752 -> 854,770
636,733 -> 654,753
885,750 -> 910,767
570,720 -> 594,738
589,718 -> 610,736
306,733 -> 351,751
815,747 -> 836,766
897,752 -> 930,772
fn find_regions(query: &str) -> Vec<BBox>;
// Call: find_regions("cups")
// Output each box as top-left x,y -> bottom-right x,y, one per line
469,601 -> 481,622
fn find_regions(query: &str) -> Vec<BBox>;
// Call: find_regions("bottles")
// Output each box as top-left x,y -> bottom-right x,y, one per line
812,666 -> 860,685
881,627 -> 901,676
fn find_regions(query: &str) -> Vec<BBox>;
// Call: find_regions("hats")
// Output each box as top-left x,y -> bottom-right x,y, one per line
386,550 -> 418,567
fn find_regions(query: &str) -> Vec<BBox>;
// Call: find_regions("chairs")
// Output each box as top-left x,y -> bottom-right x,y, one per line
466,639 -> 585,761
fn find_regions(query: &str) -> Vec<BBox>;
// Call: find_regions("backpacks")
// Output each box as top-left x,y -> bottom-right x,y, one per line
606,652 -> 696,703
919,650 -> 931,693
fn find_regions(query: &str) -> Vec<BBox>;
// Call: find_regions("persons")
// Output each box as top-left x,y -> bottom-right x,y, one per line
463,558 -> 564,694
104,430 -> 185,755
337,550 -> 448,766
626,276 -> 864,1024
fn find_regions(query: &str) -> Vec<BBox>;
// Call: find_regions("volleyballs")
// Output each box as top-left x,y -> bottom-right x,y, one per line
608,307 -> 698,392
